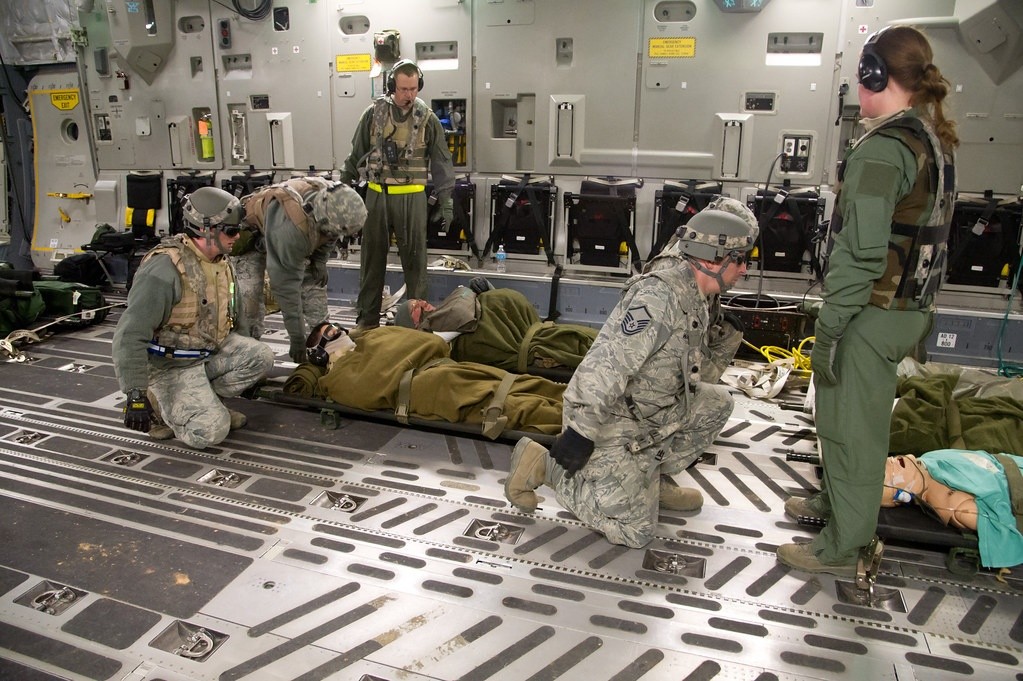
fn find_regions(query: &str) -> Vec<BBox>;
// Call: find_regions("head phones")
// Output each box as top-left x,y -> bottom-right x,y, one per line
857,26 -> 893,93
304,345 -> 330,367
387,58 -> 424,93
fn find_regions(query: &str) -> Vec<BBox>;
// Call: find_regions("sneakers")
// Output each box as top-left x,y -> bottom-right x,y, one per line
346,321 -> 380,341
652,475 -> 706,512
503,436 -> 550,514
226,407 -> 248,431
147,408 -> 175,440
775,541 -> 859,577
783,493 -> 833,525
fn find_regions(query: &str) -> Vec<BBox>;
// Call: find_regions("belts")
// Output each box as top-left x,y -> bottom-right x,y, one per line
366,180 -> 426,195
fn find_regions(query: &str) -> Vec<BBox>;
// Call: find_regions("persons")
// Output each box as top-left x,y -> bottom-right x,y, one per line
505,195 -> 760,549
227,176 -> 368,363
305,321 -> 569,436
394,277 -> 601,380
338,59 -> 456,330
776,27 -> 957,583
112,187 -> 274,450
890,357 -> 1023,456
881,448 -> 1023,531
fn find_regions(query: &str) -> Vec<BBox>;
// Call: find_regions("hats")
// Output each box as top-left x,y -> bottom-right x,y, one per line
700,197 -> 760,250
392,301 -> 414,329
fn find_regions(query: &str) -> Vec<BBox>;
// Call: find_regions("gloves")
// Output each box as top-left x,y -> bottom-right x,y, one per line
123,395 -> 155,433
288,336 -> 308,364
809,318 -> 842,386
470,276 -> 490,296
430,198 -> 455,232
548,425 -> 594,480
796,300 -> 822,318
721,310 -> 746,333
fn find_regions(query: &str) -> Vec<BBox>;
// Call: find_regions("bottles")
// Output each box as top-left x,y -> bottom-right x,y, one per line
497,244 -> 506,275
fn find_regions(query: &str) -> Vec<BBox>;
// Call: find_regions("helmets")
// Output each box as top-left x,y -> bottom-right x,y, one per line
180,187 -> 249,225
677,210 -> 752,263
313,178 -> 373,237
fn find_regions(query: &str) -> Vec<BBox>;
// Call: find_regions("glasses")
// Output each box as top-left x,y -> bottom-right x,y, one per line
727,251 -> 748,265
410,298 -> 423,314
322,322 -> 349,340
395,85 -> 419,93
212,224 -> 242,237
